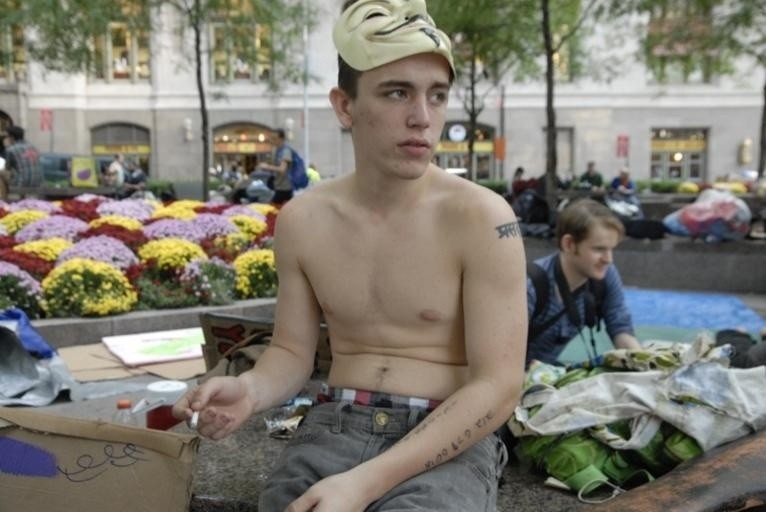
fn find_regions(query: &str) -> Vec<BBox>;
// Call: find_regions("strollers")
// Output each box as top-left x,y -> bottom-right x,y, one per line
512,189 -> 552,239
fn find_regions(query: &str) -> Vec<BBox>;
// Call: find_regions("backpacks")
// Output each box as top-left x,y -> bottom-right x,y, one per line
277,146 -> 308,189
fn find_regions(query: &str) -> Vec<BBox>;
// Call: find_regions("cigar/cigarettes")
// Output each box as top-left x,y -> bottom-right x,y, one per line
190,409 -> 200,429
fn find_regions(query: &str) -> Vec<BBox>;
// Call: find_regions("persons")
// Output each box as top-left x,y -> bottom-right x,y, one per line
257,127 -> 295,206
102,151 -> 324,191
516,200 -> 641,371
0,126 -> 44,204
169,2 -> 533,511
513,167 -> 525,182
580,161 -> 605,192
612,168 -> 645,217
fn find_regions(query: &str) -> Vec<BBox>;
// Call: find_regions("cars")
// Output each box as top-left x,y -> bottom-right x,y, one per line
38,154 -> 144,183
